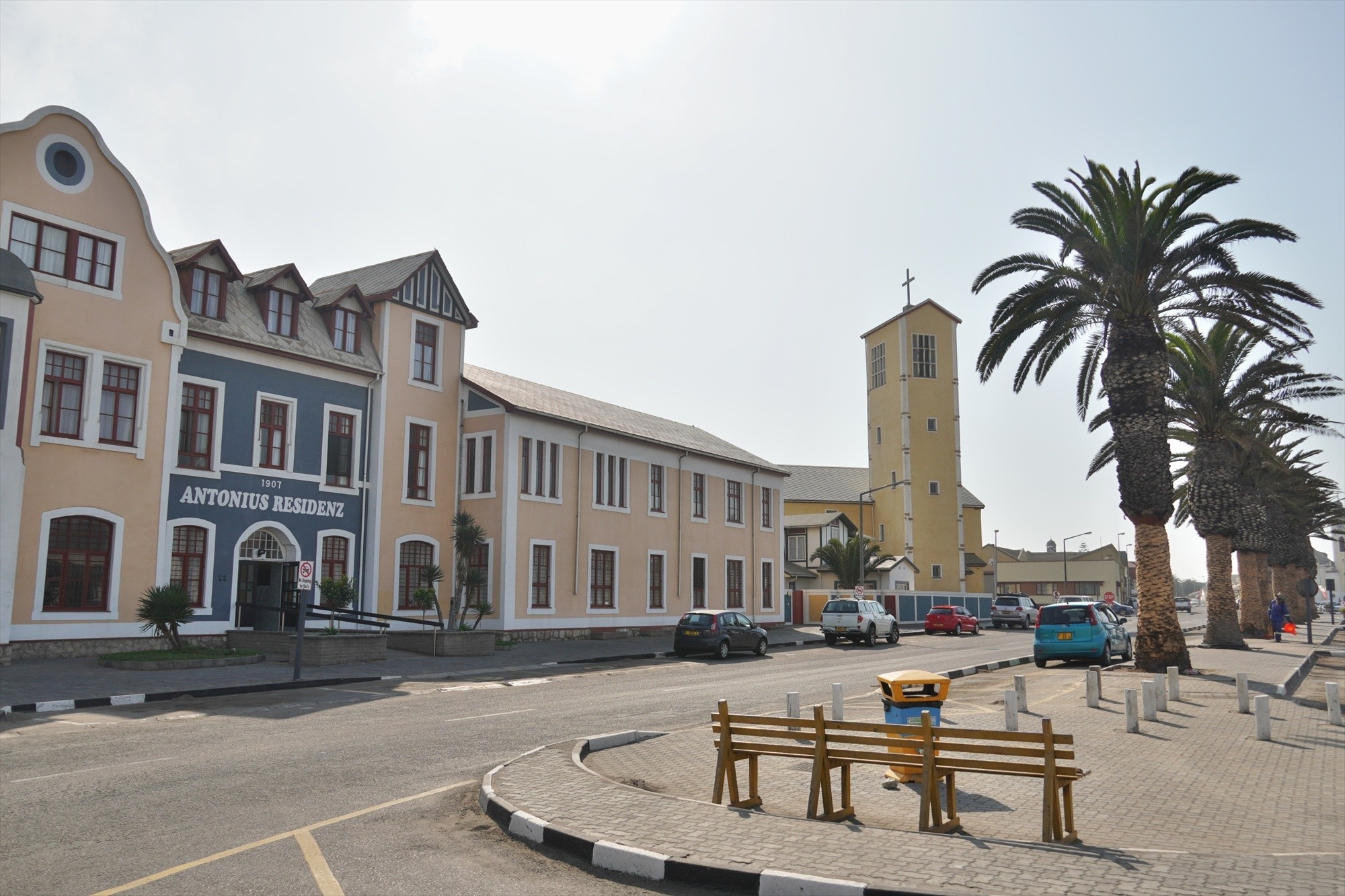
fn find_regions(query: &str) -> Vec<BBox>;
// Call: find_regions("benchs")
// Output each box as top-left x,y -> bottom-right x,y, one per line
711,699 -> 1093,846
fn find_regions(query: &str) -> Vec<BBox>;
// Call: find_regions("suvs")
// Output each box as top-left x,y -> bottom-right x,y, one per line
819,597 -> 899,647
989,591 -> 1039,630
1175,596 -> 1192,613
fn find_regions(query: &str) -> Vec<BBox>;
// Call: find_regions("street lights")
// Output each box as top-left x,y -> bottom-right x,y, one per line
994,529 -> 999,598
1062,531 -> 1093,595
858,479 -> 912,598
1117,532 -> 1125,604
1125,544 -> 1132,601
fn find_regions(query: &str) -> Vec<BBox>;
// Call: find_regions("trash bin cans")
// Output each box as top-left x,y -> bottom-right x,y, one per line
877,670 -> 951,782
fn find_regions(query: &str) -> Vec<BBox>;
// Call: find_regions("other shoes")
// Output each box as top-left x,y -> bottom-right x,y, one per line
1276,634 -> 1282,643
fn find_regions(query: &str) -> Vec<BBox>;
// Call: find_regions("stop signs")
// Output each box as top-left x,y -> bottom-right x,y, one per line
1103,591 -> 1115,602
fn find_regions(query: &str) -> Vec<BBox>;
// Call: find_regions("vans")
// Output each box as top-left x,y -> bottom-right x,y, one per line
1057,596 -> 1095,603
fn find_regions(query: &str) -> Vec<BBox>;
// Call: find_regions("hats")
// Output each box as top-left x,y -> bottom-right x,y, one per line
1275,592 -> 1285,600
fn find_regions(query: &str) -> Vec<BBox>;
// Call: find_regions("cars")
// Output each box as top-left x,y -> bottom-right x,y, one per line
925,606 -> 980,636
1033,601 -> 1133,668
1190,599 -> 1198,605
1095,599 -> 1135,617
1319,601 -> 1340,612
673,610 -> 768,657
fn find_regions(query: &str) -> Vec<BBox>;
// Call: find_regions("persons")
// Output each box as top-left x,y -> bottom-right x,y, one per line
1129,598 -> 1134,608
1268,592 -> 1292,642
